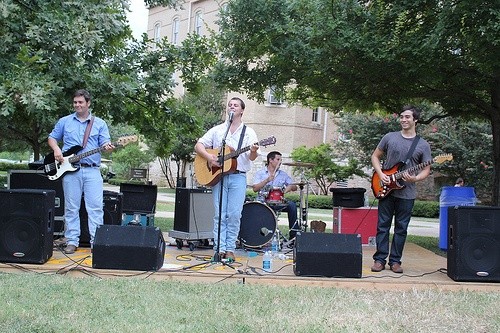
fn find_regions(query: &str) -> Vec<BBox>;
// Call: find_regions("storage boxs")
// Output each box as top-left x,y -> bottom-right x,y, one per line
332,206 -> 378,246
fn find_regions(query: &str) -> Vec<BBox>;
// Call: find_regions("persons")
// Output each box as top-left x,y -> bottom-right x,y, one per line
372,106 -> 431,273
455,178 -> 464,187
48,90 -> 114,254
195,97 -> 299,264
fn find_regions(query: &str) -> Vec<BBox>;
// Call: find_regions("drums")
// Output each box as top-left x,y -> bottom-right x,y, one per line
237,200 -> 278,248
260,186 -> 285,205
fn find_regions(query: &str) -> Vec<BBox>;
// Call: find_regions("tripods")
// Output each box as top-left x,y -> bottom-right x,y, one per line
182,120 -> 236,269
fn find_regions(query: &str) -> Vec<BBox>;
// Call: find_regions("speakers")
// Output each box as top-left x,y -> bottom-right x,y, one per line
78,183 -> 166,271
174,187 -> 215,233
0,189 -> 57,264
292,232 -> 362,278
447,205 -> 500,283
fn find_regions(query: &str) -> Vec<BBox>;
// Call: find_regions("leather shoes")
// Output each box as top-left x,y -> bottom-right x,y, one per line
371,262 -> 385,272
390,263 -> 403,273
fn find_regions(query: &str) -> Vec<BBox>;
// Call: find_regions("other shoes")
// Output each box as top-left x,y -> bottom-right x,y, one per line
289,240 -> 295,245
218,251 -> 235,261
64,245 -> 77,254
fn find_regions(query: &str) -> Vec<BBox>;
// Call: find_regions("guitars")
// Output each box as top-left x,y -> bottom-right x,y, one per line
194,135 -> 276,187
43,134 -> 139,181
371,152 -> 453,200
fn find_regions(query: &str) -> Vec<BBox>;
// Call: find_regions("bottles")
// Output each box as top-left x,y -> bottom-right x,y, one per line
272,237 -> 278,257
263,249 -> 270,272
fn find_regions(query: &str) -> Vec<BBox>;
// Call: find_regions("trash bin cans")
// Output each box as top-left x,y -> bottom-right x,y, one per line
177,177 -> 186,187
439,187 -> 477,249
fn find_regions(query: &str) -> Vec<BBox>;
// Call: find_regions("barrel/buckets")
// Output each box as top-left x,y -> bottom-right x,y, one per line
437,186 -> 476,249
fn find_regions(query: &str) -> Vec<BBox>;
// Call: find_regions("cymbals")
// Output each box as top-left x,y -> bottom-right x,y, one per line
287,182 -> 312,185
282,162 -> 315,166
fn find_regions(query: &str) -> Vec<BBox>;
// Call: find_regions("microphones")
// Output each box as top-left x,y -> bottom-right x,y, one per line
229,111 -> 234,122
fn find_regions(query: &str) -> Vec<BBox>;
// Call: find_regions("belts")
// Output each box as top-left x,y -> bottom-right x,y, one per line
75,163 -> 96,167
233,171 -> 246,174
